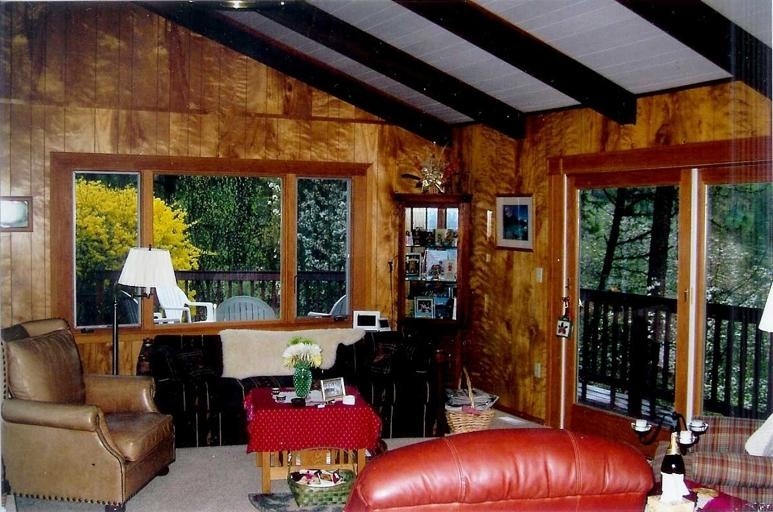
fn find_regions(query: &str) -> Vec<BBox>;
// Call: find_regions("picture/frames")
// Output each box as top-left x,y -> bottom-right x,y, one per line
352,310 -> 380,329
0,196 -> 33,232
413,295 -> 436,318
494,194 -> 536,253
319,377 -> 346,403
404,253 -> 421,281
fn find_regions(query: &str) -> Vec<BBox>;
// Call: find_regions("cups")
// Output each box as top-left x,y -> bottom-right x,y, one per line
276,396 -> 287,401
271,388 -> 280,395
439,271 -> 456,280
291,398 -> 305,408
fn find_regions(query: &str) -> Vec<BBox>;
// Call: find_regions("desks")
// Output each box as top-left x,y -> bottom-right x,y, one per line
646,478 -> 759,512
244,386 -> 383,493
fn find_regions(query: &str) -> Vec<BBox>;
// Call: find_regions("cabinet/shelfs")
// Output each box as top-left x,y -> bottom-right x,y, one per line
397,191 -> 472,390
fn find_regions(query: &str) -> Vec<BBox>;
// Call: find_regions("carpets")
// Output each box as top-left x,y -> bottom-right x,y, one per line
247,493 -> 347,512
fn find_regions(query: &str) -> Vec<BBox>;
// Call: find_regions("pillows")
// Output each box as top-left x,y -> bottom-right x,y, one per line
168,346 -> 213,380
374,342 -> 415,369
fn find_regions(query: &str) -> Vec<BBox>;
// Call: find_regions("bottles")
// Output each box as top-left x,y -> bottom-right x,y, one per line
659,432 -> 686,497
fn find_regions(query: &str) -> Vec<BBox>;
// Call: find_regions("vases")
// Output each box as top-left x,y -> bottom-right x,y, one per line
294,365 -> 312,397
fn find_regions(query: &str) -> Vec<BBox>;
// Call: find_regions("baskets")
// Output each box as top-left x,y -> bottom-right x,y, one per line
445,366 -> 500,434
287,446 -> 360,509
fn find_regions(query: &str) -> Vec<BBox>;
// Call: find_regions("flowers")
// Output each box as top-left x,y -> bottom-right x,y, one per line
282,335 -> 322,368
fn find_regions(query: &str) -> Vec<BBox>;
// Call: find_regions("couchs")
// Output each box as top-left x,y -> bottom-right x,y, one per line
136,330 -> 437,447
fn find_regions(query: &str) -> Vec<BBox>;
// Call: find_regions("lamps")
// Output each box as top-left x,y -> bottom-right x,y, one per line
112,247 -> 177,302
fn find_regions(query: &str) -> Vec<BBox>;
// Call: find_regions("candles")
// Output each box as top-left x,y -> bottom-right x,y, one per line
634,418 -> 705,445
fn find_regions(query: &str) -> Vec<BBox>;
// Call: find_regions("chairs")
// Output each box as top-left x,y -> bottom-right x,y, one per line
216,295 -> 278,321
342,429 -> 654,512
653,416 -> 773,504
0,318 -> 176,512
156,283 -> 214,323
308,293 -> 347,319
119,290 -> 179,324
